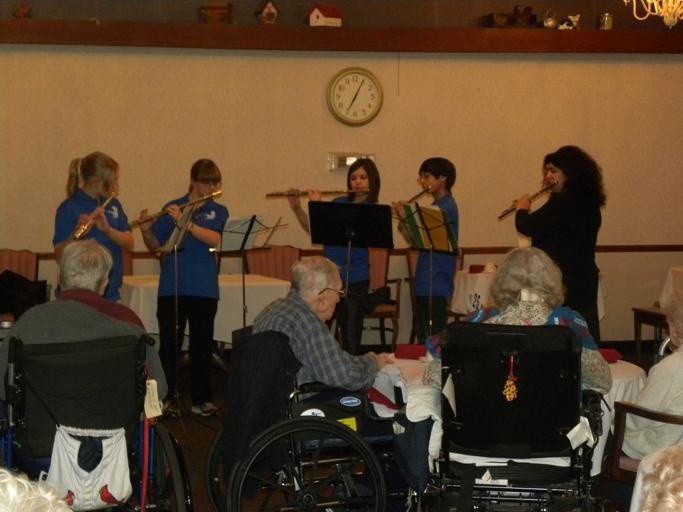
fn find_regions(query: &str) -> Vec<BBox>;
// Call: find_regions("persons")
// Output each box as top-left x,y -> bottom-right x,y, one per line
139,159 -> 231,418
0,466 -> 74,512
423,247 -> 614,490
607,266 -> 683,461
392,158 -> 458,344
1,238 -> 168,404
285,157 -> 380,356
629,449 -> 683,512
251,255 -> 399,498
53,151 -> 135,301
515,145 -> 606,344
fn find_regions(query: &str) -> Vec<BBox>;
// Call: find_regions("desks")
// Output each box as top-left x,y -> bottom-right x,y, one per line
374,358 -> 645,476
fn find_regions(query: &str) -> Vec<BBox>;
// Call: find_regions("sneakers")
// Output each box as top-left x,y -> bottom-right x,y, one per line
161,401 -> 178,418
191,402 -> 220,417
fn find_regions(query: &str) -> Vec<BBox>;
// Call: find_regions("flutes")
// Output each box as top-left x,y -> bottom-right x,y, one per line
73,191 -> 119,240
130,190 -> 224,227
405,184 -> 434,205
265,189 -> 373,197
497,180 -> 558,221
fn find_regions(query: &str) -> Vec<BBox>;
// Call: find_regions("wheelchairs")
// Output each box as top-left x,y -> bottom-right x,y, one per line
206,325 -> 441,511
2,337 -> 193,511
436,324 -> 604,512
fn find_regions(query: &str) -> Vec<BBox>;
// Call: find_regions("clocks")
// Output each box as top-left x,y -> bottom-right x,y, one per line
325,68 -> 384,127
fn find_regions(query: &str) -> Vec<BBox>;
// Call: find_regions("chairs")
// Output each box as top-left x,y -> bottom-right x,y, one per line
600,402 -> 683,501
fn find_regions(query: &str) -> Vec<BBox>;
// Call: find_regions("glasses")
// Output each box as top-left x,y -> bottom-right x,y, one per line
318,288 -> 346,299
